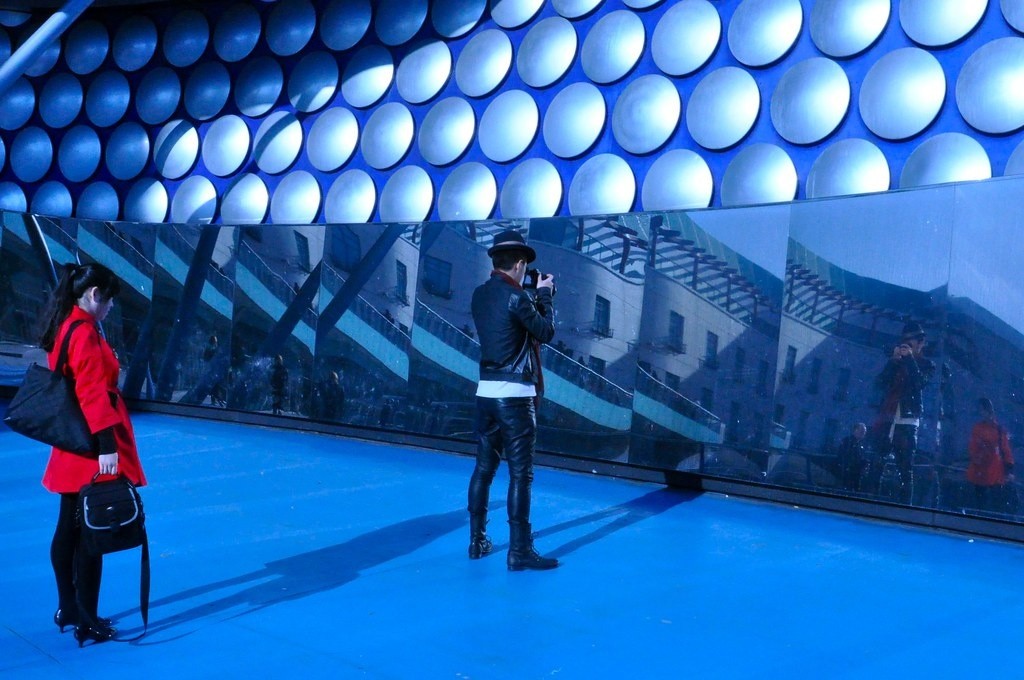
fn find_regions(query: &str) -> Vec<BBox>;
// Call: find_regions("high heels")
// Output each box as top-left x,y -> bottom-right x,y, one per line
54,609 -> 112,633
74,625 -> 117,647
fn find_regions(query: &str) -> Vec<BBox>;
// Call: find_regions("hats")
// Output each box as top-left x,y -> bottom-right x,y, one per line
487,230 -> 536,263
900,323 -> 926,341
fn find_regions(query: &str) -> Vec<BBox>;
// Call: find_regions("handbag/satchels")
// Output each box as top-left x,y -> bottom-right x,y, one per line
75,470 -> 142,555
1006,480 -> 1021,514
3,322 -> 117,459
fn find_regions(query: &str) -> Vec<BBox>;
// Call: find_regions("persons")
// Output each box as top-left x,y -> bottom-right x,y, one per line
175,333 -> 393,427
958,398 -> 1014,512
468,231 -> 560,571
835,422 -> 867,491
37,261 -> 148,648
863,322 -> 935,506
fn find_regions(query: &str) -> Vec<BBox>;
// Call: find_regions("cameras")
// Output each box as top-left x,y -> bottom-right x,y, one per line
528,268 -> 558,296
896,343 -> 913,359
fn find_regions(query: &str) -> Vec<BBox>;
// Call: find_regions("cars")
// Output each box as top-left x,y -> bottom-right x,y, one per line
375,395 -> 478,438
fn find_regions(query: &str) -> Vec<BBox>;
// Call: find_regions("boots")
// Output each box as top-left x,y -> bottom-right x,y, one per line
469,512 -> 493,558
869,474 -> 882,499
897,485 -> 913,506
507,523 -> 559,571
